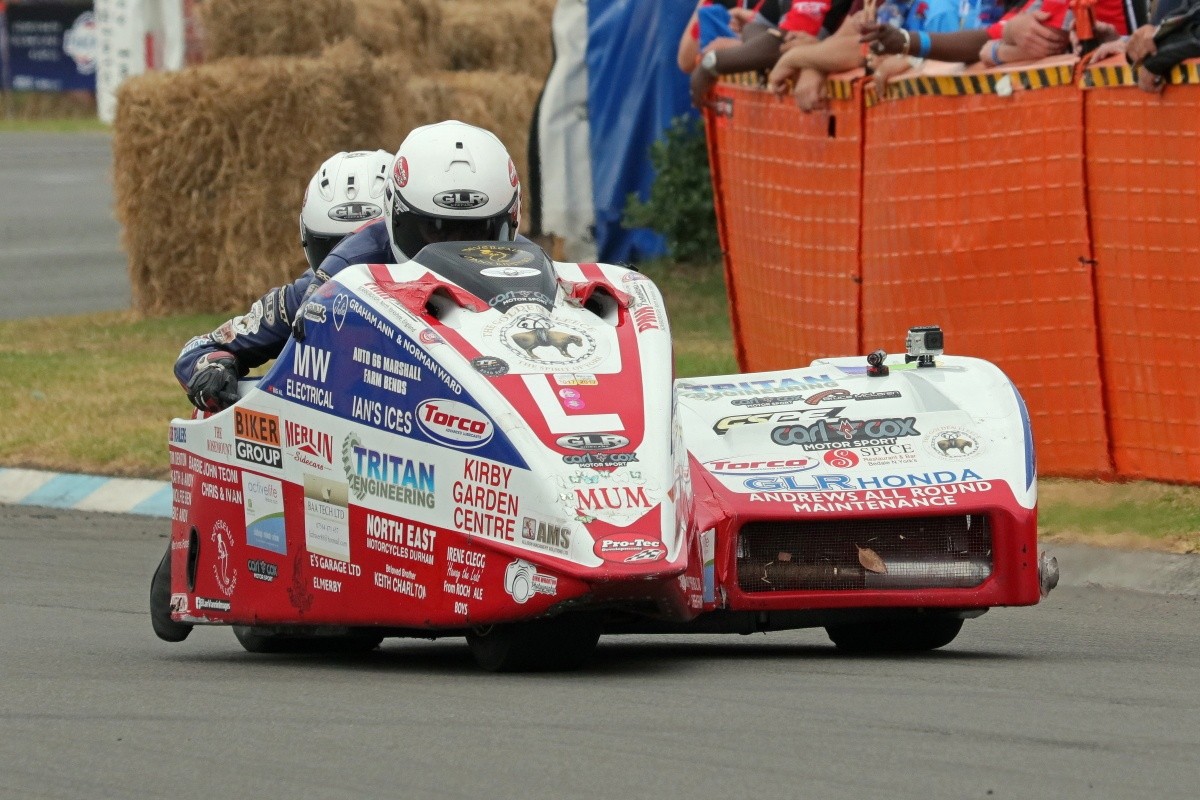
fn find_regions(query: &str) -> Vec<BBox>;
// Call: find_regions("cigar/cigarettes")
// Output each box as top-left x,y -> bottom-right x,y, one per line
875,16 -> 883,32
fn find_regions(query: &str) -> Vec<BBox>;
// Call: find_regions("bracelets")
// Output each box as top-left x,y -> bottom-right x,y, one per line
898,27 -> 911,55
917,30 -> 931,58
1120,35 -> 1130,42
991,40 -> 1003,65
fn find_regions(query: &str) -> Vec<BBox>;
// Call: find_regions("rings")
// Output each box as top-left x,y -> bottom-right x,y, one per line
877,42 -> 884,53
689,90 -> 693,97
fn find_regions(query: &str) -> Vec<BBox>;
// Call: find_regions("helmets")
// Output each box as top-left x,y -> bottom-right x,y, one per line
299,149 -> 395,274
382,120 -> 522,264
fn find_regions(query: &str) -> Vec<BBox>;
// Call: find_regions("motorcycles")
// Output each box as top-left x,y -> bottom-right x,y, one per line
150,241 -> 1060,681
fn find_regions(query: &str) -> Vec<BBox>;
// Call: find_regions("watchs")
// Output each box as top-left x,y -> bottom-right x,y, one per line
700,50 -> 723,77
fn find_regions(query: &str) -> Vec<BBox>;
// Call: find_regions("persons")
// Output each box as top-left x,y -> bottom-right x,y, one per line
677,0 -> 1200,116
292,120 -> 540,347
173,148 -> 395,413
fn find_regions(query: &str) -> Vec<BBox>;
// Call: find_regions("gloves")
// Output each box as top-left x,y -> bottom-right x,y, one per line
186,352 -> 241,412
